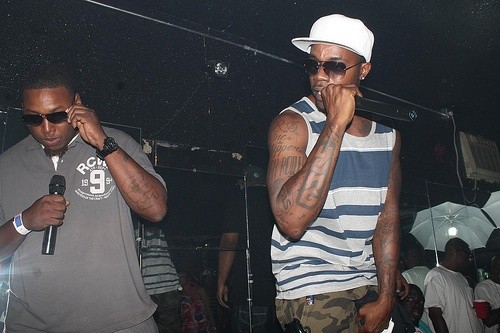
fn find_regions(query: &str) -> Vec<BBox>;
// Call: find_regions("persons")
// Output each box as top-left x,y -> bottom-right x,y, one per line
133,166 -> 500,333
0,70 -> 168,333
266,14 -> 404,333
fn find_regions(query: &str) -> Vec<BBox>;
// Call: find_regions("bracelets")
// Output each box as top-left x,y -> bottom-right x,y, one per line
13,212 -> 32,235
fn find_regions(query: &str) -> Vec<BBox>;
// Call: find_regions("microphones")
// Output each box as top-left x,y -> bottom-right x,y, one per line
354,95 -> 417,122
42,175 -> 66,255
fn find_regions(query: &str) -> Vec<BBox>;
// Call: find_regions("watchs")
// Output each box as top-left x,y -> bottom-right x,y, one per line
96,136 -> 119,160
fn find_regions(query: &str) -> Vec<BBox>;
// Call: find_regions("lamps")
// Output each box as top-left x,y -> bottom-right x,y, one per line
192,36 -> 228,77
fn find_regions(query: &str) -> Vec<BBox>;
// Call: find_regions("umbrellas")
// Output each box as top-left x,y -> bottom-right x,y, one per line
481,190 -> 500,228
409,201 -> 494,253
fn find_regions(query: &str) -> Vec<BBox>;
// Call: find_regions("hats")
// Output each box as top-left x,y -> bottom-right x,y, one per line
291,13 -> 375,63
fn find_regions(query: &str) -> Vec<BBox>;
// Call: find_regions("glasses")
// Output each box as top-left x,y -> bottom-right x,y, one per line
304,58 -> 365,80
20,97 -> 75,126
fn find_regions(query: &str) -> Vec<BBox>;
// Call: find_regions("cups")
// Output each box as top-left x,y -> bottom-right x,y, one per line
473,299 -> 490,319
477,268 -> 485,282
484,272 -> 490,279
